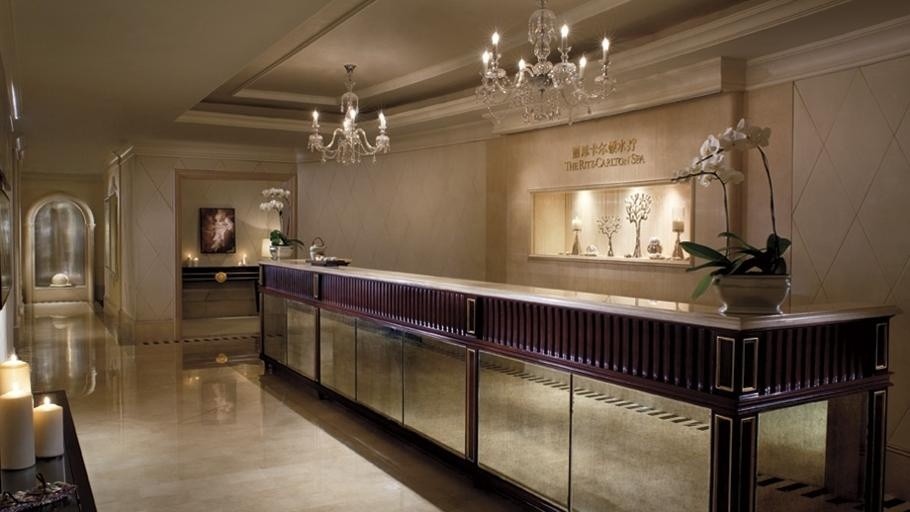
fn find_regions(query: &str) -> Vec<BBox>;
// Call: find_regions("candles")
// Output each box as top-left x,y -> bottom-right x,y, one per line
672,221 -> 684,232
0,359 -> 66,471
572,219 -> 582,231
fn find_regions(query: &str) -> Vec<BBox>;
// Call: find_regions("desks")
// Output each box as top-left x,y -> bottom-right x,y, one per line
1,387 -> 103,511
181,266 -> 260,312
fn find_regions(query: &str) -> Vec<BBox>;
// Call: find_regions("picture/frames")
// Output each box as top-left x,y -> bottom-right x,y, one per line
199,206 -> 237,254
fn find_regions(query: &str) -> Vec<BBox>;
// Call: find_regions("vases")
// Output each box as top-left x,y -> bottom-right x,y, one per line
711,277 -> 790,318
271,246 -> 301,262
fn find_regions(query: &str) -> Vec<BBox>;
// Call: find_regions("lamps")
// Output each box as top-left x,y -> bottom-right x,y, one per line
471,0 -> 617,130
306,60 -> 392,163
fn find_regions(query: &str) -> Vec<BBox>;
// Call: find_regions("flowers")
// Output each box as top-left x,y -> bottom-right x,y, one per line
670,116 -> 793,303
260,184 -> 306,255
